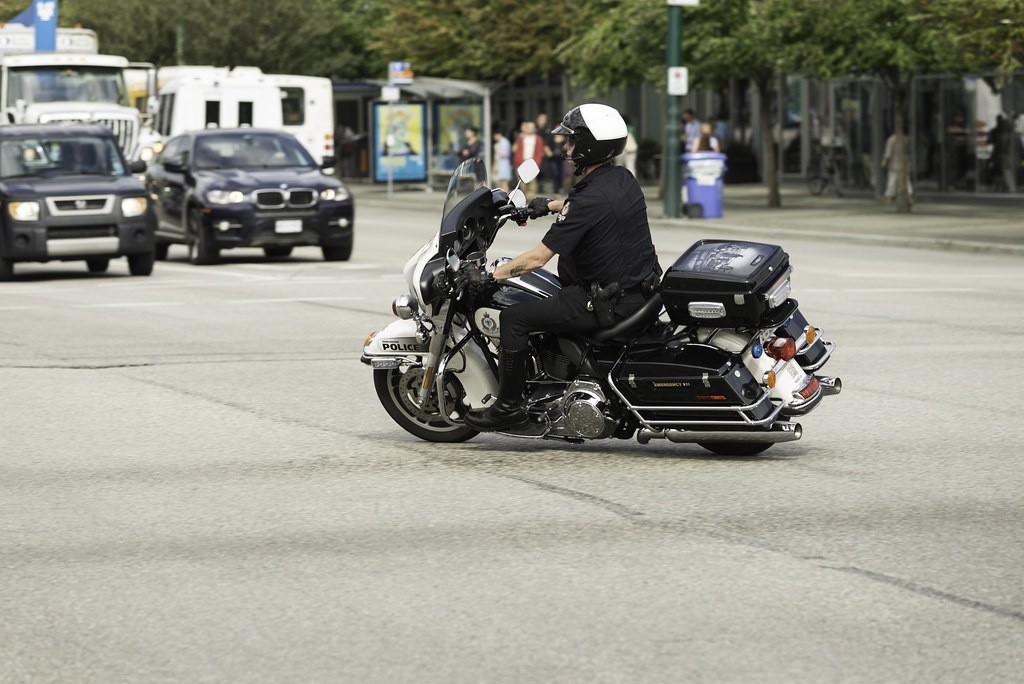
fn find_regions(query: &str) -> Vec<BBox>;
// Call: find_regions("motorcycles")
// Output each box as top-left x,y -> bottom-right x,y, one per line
358,157 -> 842,457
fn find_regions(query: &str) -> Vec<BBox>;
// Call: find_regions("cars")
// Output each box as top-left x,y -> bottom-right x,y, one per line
0,122 -> 156,277
148,128 -> 355,266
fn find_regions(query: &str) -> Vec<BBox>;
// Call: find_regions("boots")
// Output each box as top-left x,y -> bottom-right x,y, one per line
464,348 -> 529,434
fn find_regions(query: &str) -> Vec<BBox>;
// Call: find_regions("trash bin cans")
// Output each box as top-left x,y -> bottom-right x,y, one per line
679,152 -> 727,219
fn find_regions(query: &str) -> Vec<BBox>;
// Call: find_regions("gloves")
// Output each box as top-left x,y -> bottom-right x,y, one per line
454,268 -> 497,296
527,196 -> 553,220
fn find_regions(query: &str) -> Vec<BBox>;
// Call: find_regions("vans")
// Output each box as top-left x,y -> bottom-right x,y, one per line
264,73 -> 338,175
151,65 -> 283,149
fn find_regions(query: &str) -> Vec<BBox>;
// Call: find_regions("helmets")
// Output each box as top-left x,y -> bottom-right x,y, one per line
552,102 -> 627,176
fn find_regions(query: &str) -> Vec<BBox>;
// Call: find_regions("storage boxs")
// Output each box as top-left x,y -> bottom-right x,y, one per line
659,238 -> 794,328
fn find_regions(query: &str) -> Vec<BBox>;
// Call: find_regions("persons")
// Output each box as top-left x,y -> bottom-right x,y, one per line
988,114 -> 1024,181
881,128 -> 914,203
493,114 -> 574,196
684,110 -> 734,181
459,104 -> 663,432
459,128 -> 479,161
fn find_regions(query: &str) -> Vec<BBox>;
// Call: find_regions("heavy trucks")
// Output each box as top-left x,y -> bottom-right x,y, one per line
0,0 -> 167,168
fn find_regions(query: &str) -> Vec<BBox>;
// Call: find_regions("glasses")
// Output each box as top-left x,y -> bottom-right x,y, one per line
566,135 -> 575,147
